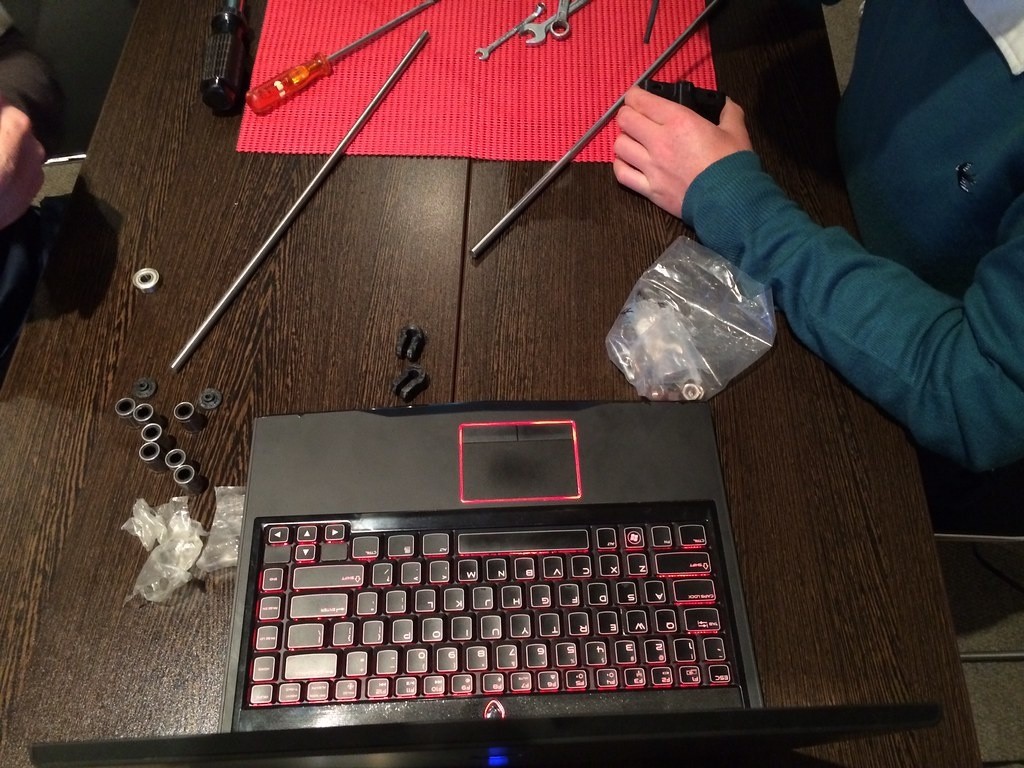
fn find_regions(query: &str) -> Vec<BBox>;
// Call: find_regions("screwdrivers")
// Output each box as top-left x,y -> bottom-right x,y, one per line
199,0 -> 251,110
244,0 -> 442,115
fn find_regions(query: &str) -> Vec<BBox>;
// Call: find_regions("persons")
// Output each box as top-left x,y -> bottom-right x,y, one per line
0,0 -> 66,232
612,0 -> 1024,474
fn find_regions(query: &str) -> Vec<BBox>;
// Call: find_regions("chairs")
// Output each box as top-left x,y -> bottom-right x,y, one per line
933,504 -> 1024,663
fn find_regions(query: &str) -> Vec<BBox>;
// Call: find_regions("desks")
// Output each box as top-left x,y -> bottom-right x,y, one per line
0,0 -> 985,768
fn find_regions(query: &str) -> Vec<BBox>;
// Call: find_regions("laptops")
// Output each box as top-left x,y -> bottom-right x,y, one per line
26,399 -> 945,768
